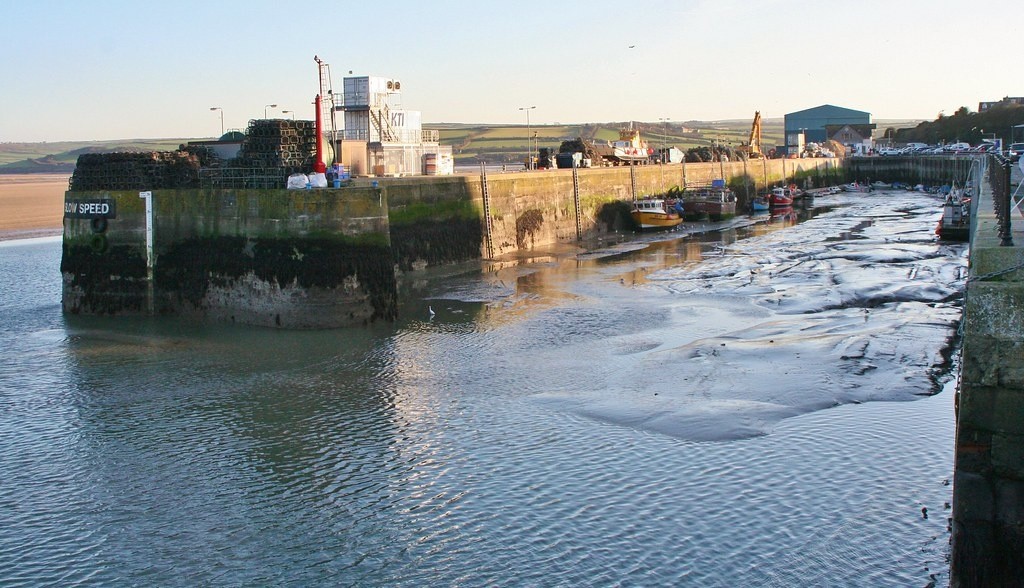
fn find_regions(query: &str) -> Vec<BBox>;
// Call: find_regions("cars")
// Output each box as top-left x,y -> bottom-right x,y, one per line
1009,142 -> 1024,161
880,146 -> 967,156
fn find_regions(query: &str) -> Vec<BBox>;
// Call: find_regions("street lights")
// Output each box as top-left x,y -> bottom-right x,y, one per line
264,104 -> 277,117
659,117 -> 670,164
210,106 -> 224,137
282,110 -> 295,120
798,128 -> 808,134
518,106 -> 536,171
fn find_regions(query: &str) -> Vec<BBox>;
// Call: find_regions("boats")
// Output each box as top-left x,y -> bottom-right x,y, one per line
872,179 -> 942,194
631,178 -> 737,230
600,127 -> 650,161
750,182 -> 874,210
935,160 -> 974,241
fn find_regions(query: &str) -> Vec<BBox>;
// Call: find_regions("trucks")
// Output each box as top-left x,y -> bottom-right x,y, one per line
906,142 -> 928,148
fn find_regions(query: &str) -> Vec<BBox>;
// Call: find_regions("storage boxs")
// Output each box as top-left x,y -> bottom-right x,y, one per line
788,134 -> 805,158
341,76 -> 453,178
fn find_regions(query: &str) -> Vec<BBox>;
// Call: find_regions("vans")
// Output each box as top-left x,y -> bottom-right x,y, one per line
950,142 -> 971,149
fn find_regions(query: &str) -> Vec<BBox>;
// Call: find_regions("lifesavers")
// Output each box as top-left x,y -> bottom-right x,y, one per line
90,234 -> 107,252
90,215 -> 108,233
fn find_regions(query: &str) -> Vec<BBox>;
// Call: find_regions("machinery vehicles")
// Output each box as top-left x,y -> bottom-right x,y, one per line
736,110 -> 766,160
